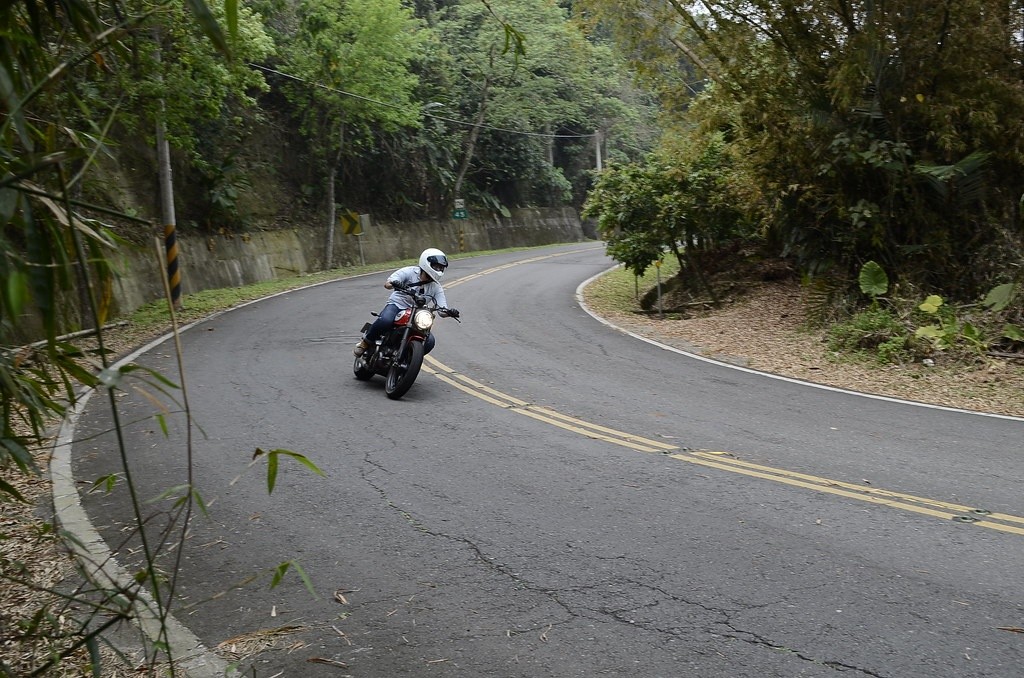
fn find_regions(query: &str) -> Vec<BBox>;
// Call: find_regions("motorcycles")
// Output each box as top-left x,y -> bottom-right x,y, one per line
352,281 -> 462,400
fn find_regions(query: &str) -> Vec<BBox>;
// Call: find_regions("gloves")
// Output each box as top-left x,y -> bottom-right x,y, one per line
390,280 -> 410,291
446,308 -> 460,318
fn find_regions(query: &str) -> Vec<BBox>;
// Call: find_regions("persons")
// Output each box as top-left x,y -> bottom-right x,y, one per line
352,248 -> 460,359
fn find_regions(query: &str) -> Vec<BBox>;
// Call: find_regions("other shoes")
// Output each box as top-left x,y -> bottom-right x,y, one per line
354,334 -> 371,357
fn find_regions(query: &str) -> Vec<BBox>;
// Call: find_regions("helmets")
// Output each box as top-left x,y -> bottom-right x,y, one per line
418,248 -> 449,284
